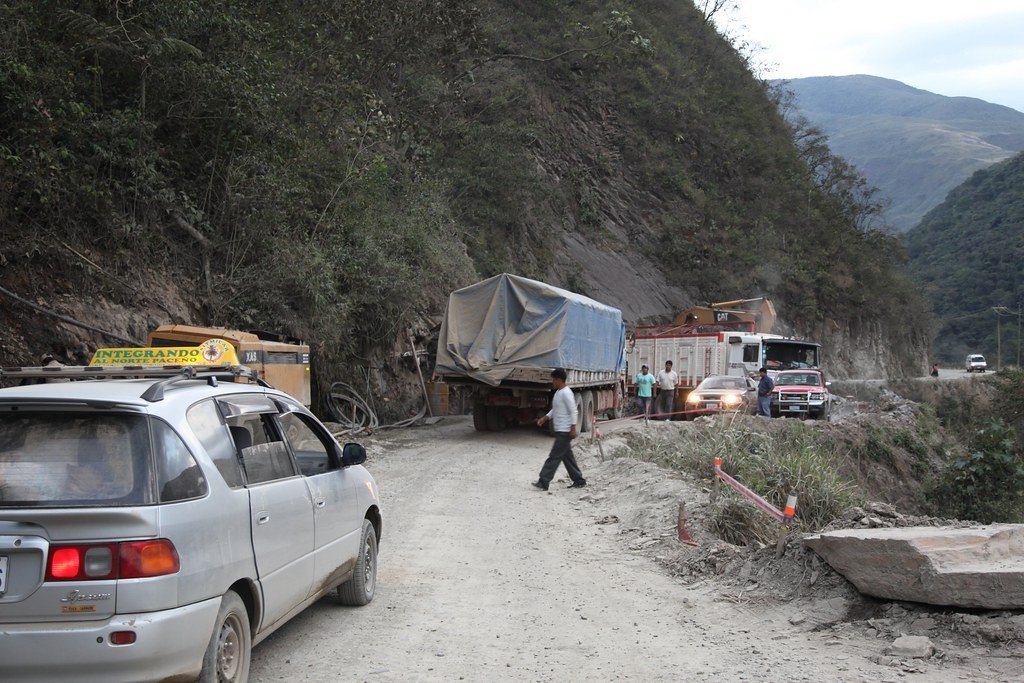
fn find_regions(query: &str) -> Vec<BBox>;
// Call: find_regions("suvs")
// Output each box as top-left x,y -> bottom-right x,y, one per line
769,367 -> 833,420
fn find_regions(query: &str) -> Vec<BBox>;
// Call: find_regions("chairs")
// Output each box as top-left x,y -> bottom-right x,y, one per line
711,381 -> 746,390
229,425 -> 267,482
783,375 -> 817,386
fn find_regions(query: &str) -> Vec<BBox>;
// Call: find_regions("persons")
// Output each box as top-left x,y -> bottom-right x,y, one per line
531,368 -> 586,491
749,372 -> 757,380
656,360 -> 679,421
757,368 -> 775,417
931,364 -> 938,376
634,365 -> 657,425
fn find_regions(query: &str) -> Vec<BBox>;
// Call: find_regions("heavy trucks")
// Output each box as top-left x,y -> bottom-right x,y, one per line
145,321 -> 313,413
625,319 -> 823,419
434,269 -> 635,436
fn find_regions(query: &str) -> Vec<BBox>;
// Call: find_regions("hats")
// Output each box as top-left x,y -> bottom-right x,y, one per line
642,364 -> 649,368
749,372 -> 757,377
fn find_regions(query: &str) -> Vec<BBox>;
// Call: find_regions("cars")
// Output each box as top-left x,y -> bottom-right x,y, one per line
684,372 -> 759,418
0,336 -> 385,683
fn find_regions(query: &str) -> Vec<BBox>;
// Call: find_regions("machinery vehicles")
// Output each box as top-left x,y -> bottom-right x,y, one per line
674,297 -> 778,333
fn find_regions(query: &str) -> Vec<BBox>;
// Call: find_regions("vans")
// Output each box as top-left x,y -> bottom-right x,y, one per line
964,353 -> 987,373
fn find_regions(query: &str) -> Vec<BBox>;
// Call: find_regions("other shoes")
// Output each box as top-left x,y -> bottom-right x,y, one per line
567,481 -> 586,489
531,481 -> 549,491
